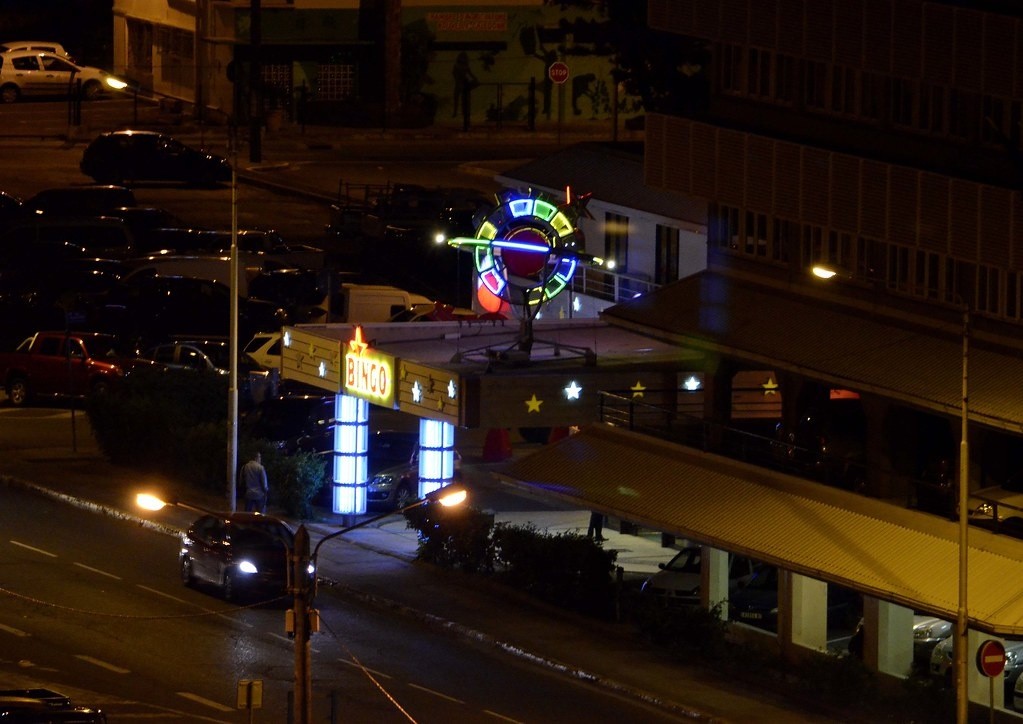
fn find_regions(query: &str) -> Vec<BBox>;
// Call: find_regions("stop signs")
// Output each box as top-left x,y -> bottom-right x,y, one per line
549,62 -> 569,84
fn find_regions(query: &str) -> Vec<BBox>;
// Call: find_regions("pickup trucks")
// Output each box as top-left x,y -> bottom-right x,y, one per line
0,331 -> 124,406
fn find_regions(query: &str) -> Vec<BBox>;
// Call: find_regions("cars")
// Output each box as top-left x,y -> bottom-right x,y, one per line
955,474 -> 1023,534
0,50 -> 106,102
0,41 -> 76,66
848,616 -> 864,658
179,512 -> 315,600
930,635 -> 953,676
913,618 -> 953,649
728,564 -> 778,629
1005,643 -> 1023,711
80,130 -> 237,185
641,547 -> 701,609
0,688 -> 107,724
324,429 -> 419,509
1,184 -> 288,333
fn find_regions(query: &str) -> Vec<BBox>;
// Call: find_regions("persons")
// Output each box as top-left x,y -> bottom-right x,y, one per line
587,511 -> 609,541
454,450 -> 463,474
410,439 -> 419,466
239,451 -> 269,513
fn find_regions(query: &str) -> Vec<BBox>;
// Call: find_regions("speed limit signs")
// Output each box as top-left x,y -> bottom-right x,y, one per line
977,640 -> 1006,677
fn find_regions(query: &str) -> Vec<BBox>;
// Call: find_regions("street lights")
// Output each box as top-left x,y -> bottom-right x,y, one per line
128,482 -> 475,724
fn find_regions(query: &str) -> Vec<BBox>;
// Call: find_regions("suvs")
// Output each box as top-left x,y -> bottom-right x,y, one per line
127,337 -> 278,406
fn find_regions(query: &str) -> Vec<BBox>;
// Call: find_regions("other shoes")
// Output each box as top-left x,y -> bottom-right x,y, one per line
595,534 -> 609,541
